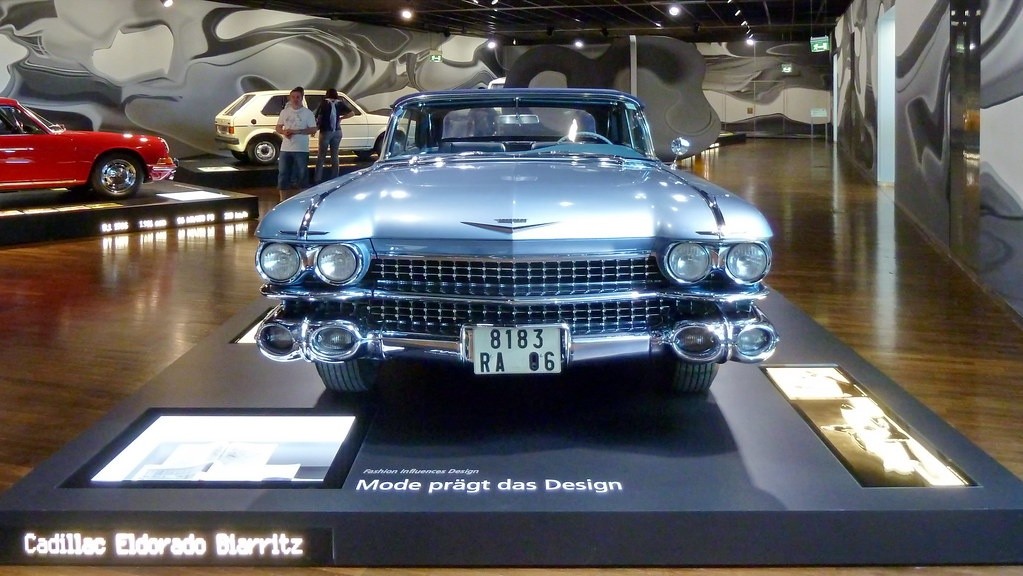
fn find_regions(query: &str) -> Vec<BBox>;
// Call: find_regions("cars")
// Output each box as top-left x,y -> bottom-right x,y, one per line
0,96 -> 180,202
252,85 -> 779,396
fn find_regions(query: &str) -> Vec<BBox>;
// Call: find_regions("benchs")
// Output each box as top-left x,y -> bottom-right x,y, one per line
421,141 -> 614,156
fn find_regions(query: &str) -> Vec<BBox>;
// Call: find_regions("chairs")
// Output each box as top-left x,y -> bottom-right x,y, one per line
531,141 -> 595,150
439,142 -> 506,153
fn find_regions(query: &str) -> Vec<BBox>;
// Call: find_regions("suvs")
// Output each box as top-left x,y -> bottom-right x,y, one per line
213,89 -> 417,167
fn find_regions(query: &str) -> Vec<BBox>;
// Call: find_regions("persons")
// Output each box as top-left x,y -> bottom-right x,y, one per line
466,107 -> 499,136
315,89 -> 354,182
275,87 -> 317,205
